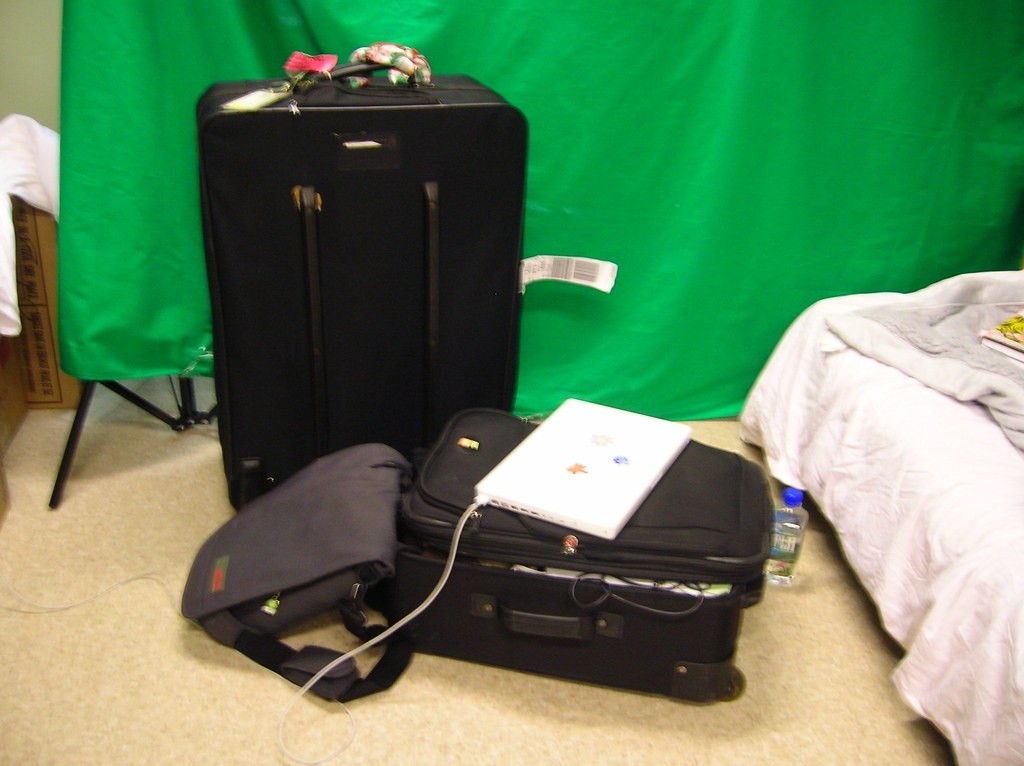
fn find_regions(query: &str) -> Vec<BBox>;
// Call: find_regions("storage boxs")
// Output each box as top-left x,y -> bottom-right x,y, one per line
0,196 -> 86,456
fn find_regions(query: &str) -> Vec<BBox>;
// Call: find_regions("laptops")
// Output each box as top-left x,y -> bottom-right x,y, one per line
474,398 -> 692,539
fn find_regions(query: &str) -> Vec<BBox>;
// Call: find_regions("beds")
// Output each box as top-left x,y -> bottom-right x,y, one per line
740,270 -> 1024,766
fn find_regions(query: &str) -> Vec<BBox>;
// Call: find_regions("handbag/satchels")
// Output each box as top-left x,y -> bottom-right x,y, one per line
179,440 -> 414,704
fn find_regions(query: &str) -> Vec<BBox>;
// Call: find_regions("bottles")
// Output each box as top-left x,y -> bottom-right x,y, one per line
764,487 -> 809,583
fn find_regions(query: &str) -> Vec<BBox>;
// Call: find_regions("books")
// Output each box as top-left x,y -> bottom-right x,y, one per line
981,308 -> 1024,363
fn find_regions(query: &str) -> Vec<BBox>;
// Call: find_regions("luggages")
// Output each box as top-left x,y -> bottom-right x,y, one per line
194,59 -> 529,513
385,404 -> 776,702
